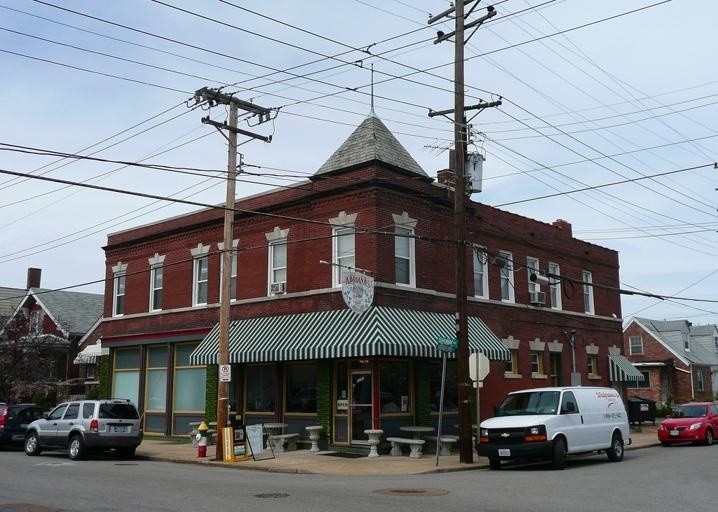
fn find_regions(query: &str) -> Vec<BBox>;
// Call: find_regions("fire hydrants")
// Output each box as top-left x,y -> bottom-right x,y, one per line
196,421 -> 209,457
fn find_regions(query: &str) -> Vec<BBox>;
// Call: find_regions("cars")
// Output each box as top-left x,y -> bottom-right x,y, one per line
0,399 -> 144,460
658,401 -> 718,447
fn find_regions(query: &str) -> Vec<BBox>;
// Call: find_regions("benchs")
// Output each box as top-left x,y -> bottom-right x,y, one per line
263,430 -> 300,452
386,434 -> 477,459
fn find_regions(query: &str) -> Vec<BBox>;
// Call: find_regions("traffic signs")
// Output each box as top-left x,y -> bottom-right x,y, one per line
435,335 -> 459,353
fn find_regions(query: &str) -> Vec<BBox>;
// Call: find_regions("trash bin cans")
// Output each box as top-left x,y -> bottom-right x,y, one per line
627,396 -> 656,424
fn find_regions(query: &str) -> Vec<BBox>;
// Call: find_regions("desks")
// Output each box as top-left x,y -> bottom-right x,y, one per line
255,422 -> 289,451
399,425 -> 435,451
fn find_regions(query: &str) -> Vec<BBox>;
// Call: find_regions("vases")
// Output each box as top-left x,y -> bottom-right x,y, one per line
364,429 -> 384,458
306,425 -> 324,451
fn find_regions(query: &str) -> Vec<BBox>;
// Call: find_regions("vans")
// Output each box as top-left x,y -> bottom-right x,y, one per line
475,386 -> 632,470
